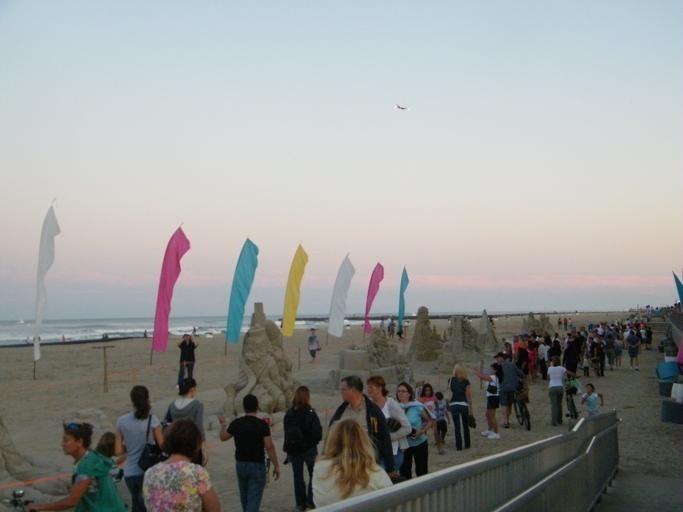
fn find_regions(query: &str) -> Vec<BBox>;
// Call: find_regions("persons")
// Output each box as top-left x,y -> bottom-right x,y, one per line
26,420 -> 128,512
311,419 -> 394,509
218,395 -> 280,512
141,419 -> 221,512
165,378 -> 205,464
480,304 -> 682,439
114,385 -> 165,512
329,362 -> 472,487
175,332 -> 199,389
306,328 -> 320,363
94,433 -> 131,512
282,385 -> 322,512
380,317 -> 397,338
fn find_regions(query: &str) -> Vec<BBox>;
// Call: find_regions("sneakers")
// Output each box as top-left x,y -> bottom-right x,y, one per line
487,431 -> 500,440
481,431 -> 494,437
500,422 -> 510,429
296,498 -> 316,511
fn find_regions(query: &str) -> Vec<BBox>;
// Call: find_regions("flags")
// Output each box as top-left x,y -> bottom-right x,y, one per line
397,268 -> 409,335
363,261 -> 384,333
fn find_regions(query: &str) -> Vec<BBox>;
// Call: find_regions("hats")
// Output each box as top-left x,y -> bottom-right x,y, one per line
493,352 -> 507,358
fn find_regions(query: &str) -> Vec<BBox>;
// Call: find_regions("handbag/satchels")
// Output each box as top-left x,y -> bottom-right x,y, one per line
486,384 -> 497,394
444,388 -> 453,402
137,444 -> 170,471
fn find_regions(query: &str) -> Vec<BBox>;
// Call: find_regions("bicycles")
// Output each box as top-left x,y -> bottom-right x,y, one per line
9,487 -> 37,512
564,375 -> 581,420
512,380 -> 531,431
